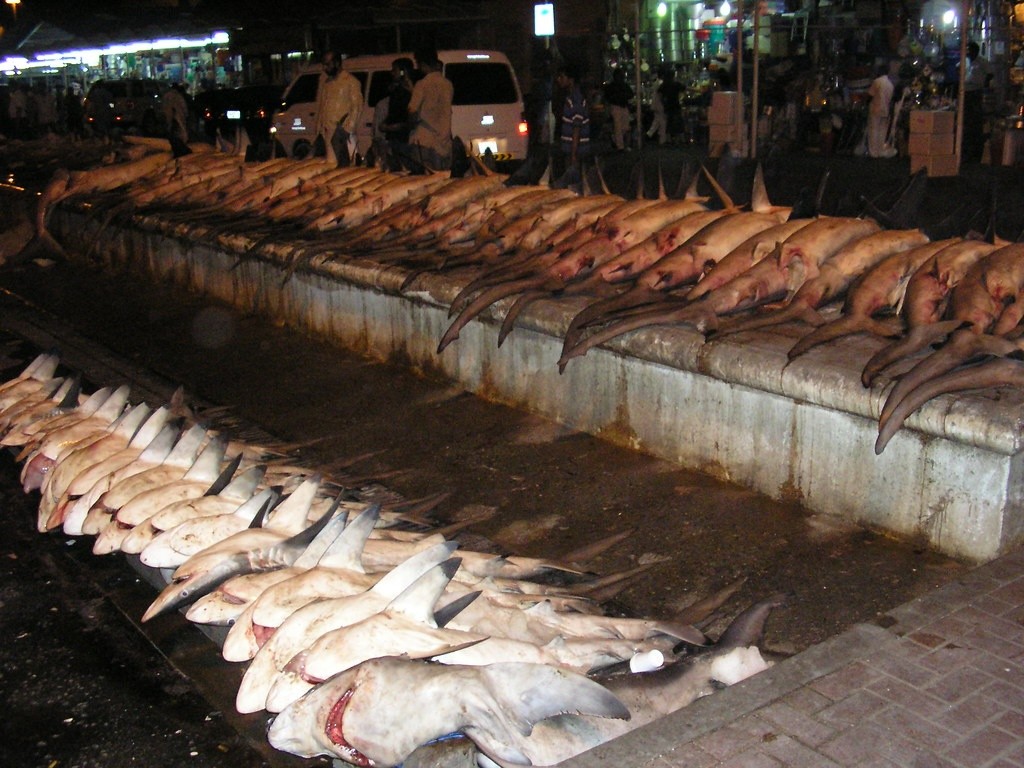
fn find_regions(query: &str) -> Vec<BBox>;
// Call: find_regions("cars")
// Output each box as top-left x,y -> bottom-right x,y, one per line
82,76 -> 284,142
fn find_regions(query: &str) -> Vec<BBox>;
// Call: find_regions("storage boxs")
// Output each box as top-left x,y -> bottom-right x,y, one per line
981,130 -> 1014,166
707,91 -> 749,159
908,111 -> 957,177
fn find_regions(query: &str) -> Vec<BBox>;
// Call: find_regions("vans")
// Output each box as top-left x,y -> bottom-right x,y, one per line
266,48 -> 529,178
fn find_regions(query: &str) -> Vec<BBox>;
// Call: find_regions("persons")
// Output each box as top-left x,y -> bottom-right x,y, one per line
0,48 -> 453,174
534,39 -> 994,186
